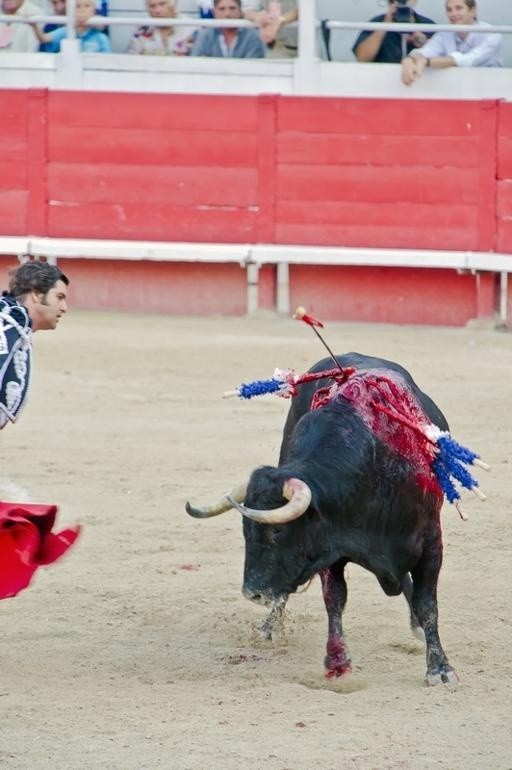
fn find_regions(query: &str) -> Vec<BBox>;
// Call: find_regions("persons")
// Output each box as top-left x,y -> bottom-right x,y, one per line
128,0 -> 299,59
1,0 -> 113,52
351,0 -> 503,86
0,258 -> 68,434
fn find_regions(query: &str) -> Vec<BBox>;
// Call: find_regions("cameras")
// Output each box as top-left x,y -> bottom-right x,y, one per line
395,7 -> 415,23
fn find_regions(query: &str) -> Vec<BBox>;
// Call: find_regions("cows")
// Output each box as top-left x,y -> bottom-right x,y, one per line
184,350 -> 458,686
